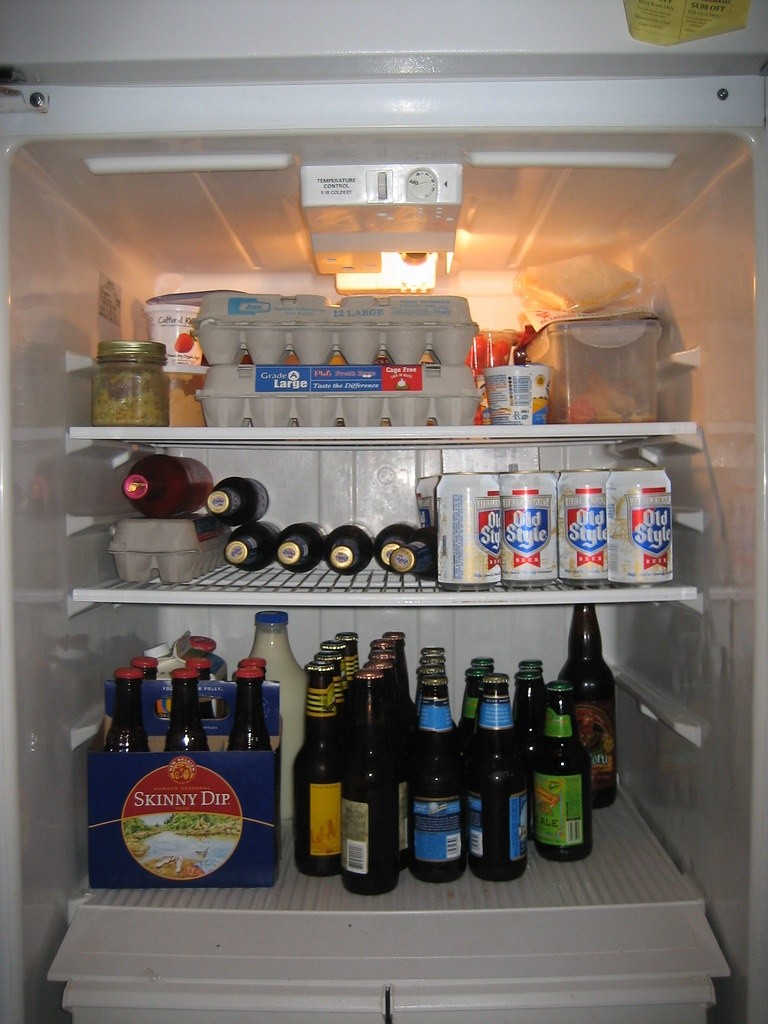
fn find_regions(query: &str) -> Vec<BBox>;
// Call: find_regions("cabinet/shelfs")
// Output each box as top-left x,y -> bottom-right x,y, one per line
69,416 -> 702,608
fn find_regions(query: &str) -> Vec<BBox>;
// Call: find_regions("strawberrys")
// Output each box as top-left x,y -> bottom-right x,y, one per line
175,330 -> 197,354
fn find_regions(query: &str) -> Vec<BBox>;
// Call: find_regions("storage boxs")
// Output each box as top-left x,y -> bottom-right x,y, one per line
516,312 -> 662,421
85,678 -> 288,889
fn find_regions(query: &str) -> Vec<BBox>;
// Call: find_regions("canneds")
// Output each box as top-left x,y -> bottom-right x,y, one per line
417,466 -> 673,589
91,340 -> 170,428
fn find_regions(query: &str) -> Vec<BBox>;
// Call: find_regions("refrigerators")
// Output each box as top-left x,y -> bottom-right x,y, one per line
0,0 -> 768,1021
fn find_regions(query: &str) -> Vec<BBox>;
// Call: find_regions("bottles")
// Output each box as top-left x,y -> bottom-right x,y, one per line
373,522 -> 421,572
224,521 -> 283,571
98,605 -> 620,895
324,522 -> 376,575
391,525 -> 442,582
88,338 -> 172,426
206,476 -> 269,526
274,522 -> 328,573
123,454 -> 215,517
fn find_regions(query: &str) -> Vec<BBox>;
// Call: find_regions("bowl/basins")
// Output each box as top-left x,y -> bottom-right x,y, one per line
144,302 -> 206,367
484,365 -> 553,425
156,362 -> 209,427
